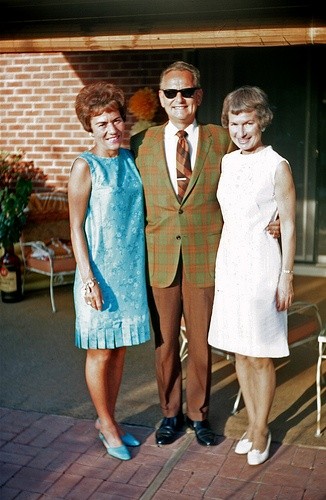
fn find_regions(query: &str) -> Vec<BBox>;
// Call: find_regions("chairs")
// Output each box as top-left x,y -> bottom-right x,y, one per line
19,210 -> 76,313
179,301 -> 326,415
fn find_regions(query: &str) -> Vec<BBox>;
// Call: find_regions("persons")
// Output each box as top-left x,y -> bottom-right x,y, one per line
207,86 -> 295,465
129,62 -> 280,445
68,85 -> 151,461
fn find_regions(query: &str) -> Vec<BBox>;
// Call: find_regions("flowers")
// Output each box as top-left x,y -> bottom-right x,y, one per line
127,87 -> 159,136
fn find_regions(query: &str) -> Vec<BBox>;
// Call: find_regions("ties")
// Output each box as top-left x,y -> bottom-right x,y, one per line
176,130 -> 192,204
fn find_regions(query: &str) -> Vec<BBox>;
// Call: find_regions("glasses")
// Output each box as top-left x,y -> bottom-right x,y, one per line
161,85 -> 199,99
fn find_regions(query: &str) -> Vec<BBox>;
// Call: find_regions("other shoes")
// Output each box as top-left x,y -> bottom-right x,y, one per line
99,431 -> 131,460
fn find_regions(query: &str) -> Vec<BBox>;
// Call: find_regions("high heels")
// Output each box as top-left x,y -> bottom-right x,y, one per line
95,418 -> 140,446
247,430 -> 271,465
235,432 -> 252,454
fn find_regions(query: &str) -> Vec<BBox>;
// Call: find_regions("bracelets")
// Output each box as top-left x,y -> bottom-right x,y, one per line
281,269 -> 293,274
82,277 -> 97,294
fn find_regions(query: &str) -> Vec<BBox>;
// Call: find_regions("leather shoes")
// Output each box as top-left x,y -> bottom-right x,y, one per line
154,412 -> 184,446
186,416 -> 216,446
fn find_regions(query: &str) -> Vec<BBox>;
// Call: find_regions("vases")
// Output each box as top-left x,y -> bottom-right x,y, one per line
0,239 -> 22,304
